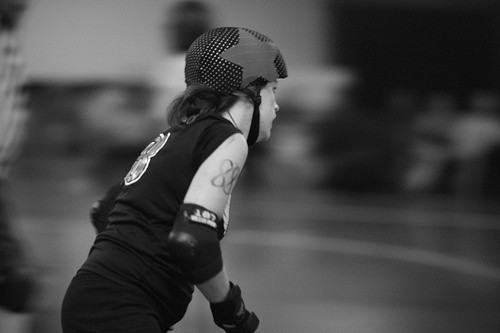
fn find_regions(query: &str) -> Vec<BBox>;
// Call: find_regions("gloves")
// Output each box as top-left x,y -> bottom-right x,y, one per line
209,280 -> 260,333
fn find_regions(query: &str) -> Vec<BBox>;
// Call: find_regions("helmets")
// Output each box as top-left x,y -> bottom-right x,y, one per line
184,25 -> 288,95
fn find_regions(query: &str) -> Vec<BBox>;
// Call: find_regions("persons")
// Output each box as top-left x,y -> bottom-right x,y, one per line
0,0 -> 44,315
60,25 -> 288,333
